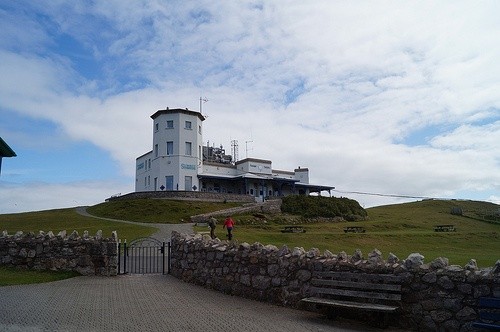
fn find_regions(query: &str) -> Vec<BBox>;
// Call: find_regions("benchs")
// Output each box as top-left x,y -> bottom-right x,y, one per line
435,224 -> 456,232
470,296 -> 500,332
302,268 -> 407,327
282,226 -> 306,234
344,226 -> 366,233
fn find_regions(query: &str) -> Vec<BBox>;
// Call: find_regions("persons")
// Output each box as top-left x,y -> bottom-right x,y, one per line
223,216 -> 234,240
209,215 -> 217,238
